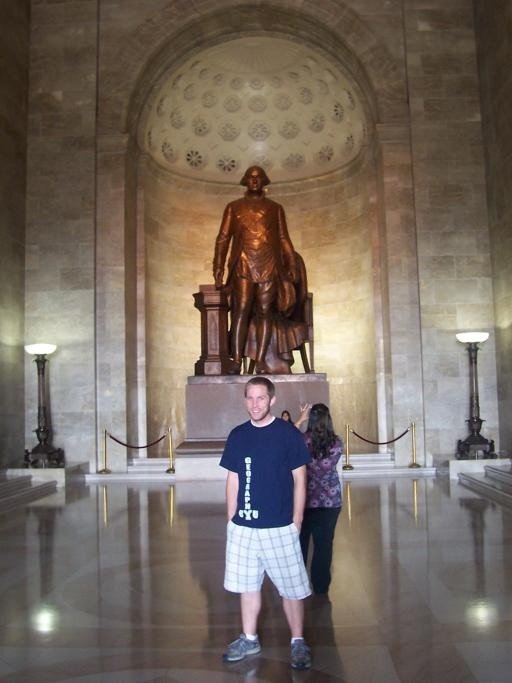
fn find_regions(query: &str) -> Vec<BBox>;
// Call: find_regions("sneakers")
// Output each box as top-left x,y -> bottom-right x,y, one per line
222,634 -> 261,661
289,637 -> 312,669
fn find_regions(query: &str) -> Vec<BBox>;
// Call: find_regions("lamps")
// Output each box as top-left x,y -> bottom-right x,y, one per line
455,332 -> 495,459
24,343 -> 64,467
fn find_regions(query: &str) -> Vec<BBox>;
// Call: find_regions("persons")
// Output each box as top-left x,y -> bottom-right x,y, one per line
211,165 -> 299,376
295,401 -> 344,597
222,652 -> 312,683
219,376 -> 313,670
246,250 -> 314,374
280,410 -> 294,424
